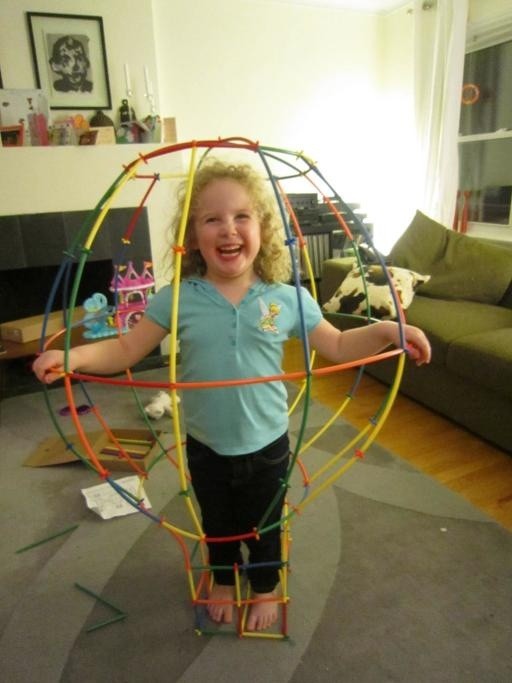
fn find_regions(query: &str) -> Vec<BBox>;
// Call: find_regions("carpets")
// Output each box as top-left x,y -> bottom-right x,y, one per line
0,363 -> 512,683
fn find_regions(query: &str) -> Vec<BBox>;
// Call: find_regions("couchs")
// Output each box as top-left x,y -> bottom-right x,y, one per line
322,237 -> 511,456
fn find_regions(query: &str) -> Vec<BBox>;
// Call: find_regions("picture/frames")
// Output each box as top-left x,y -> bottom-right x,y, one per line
26,12 -> 112,110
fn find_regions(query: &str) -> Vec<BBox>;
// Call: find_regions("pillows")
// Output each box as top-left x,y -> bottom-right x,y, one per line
323,209 -> 511,321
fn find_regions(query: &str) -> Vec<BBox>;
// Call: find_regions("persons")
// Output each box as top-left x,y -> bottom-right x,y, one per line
48,38 -> 93,94
32,160 -> 430,631
